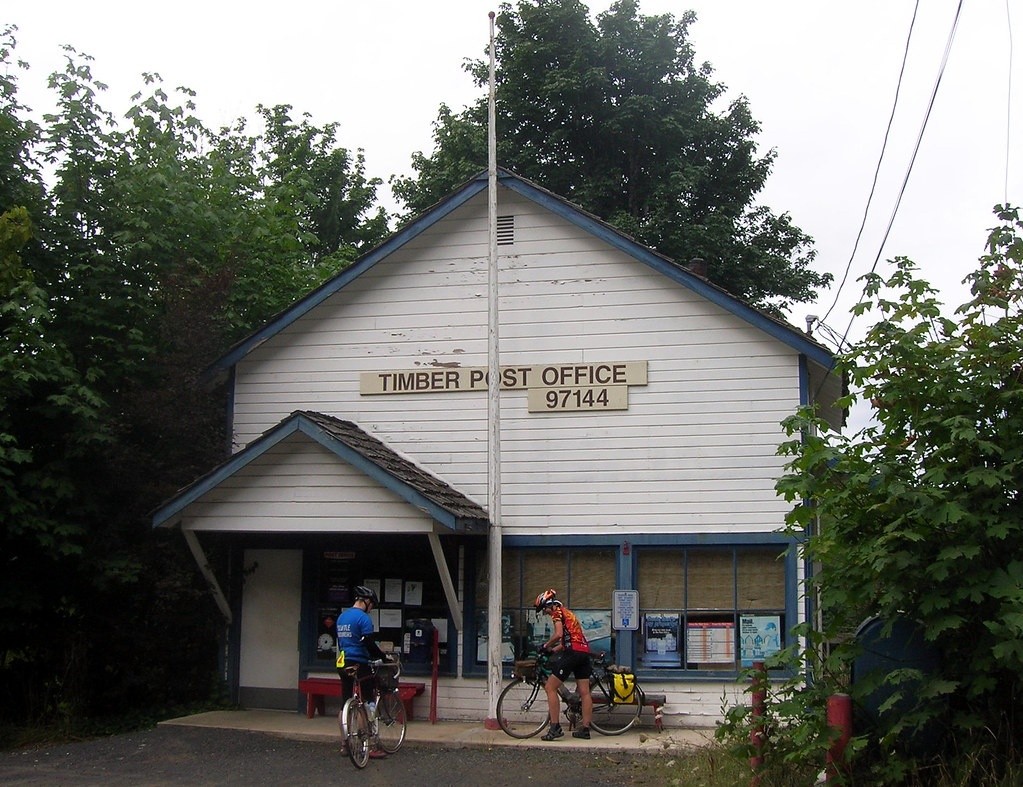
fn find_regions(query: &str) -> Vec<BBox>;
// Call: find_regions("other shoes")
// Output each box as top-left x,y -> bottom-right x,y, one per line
362,748 -> 387,759
340,745 -> 361,756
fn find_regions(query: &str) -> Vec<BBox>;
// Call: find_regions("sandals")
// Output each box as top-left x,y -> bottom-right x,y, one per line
541,726 -> 564,741
572,726 -> 591,739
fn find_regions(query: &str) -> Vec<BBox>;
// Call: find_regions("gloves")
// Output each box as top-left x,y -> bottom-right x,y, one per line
537,643 -> 546,654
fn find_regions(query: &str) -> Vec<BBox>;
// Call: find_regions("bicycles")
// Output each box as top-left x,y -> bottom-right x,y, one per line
495,642 -> 645,737
339,648 -> 410,770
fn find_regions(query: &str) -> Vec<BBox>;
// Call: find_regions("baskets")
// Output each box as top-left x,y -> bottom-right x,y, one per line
514,659 -> 537,679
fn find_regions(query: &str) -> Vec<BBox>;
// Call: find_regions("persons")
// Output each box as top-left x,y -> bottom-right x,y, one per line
764,622 -> 780,658
527,615 -> 550,640
336,586 -> 394,759
535,589 -> 593,740
648,631 -> 675,650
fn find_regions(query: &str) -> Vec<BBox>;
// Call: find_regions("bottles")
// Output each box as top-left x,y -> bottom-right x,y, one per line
363,700 -> 374,721
559,684 -> 572,700
647,637 -> 676,656
369,700 -> 376,711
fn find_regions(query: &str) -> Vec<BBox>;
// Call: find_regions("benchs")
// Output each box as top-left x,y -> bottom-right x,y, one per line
300,677 -> 425,724
561,692 -> 667,734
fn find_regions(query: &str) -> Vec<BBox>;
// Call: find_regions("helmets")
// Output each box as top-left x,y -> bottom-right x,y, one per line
534,589 -> 556,613
354,585 -> 378,607
